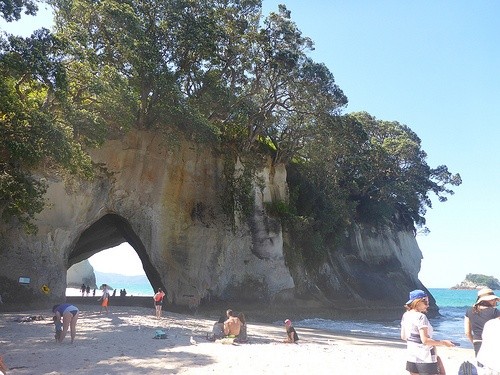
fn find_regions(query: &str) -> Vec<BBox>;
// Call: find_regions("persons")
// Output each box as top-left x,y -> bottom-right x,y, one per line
80,283 -> 96,297
112,289 -> 116,296
52,304 -> 79,344
97,284 -> 111,315
400,290 -> 455,375
283,319 -> 299,343
153,288 -> 165,318
120,289 -> 127,297
458,316 -> 500,375
209,309 -> 249,344
464,289 -> 500,368
53,316 -> 63,343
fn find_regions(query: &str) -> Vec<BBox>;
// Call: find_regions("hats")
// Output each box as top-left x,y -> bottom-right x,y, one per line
473,288 -> 500,308
405,289 -> 427,304
